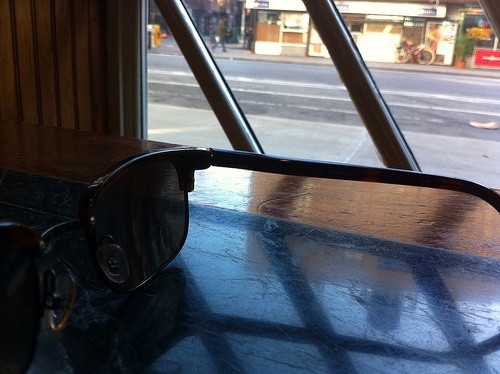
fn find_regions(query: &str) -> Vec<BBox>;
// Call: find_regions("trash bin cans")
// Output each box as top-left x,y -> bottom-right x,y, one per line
230,28 -> 240,44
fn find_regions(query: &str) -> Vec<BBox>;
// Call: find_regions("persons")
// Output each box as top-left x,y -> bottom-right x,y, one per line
210,15 -> 231,53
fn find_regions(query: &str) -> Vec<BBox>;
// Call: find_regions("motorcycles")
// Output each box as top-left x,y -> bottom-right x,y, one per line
394,39 -> 436,65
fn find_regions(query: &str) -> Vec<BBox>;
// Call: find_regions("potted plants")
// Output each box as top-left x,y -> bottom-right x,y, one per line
448,33 -> 478,69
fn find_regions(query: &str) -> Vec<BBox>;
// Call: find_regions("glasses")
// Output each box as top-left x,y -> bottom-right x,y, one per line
0,146 -> 500,373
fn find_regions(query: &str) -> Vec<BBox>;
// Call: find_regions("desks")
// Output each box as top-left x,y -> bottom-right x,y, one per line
0,117 -> 499,374
